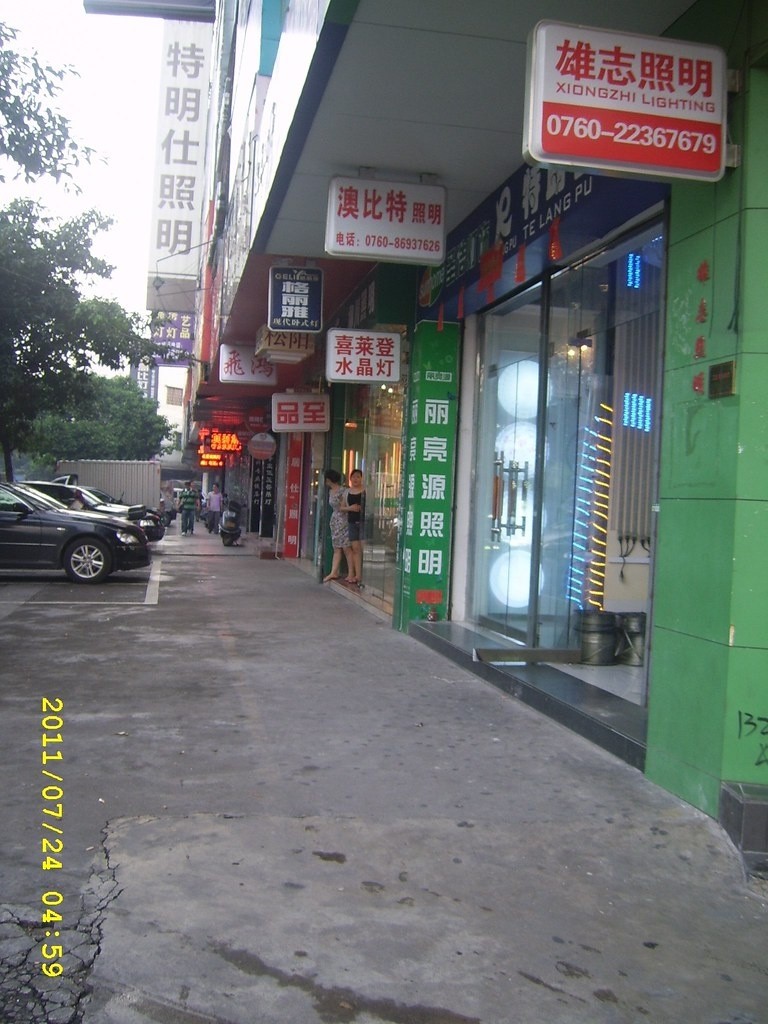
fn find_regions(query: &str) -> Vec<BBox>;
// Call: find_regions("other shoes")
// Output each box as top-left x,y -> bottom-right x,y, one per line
345,576 -> 352,581
348,576 -> 362,583
182,532 -> 187,536
323,576 -> 339,583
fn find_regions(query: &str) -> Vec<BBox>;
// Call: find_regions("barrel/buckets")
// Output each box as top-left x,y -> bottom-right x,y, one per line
570,609 -> 616,666
618,611 -> 647,666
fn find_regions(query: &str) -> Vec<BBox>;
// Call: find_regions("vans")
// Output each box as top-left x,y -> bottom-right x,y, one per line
159,488 -> 176,526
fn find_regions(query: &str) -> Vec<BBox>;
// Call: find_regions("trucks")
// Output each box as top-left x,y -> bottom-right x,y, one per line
56,459 -> 162,510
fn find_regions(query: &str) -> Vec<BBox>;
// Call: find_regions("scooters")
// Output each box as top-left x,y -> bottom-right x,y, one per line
218,499 -> 247,545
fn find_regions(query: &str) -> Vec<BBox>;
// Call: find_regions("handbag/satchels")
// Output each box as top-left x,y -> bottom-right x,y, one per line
179,505 -> 184,513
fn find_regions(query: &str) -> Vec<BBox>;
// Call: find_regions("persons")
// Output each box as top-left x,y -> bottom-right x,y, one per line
340,469 -> 365,583
177,481 -> 204,536
324,469 -> 356,582
206,482 -> 223,534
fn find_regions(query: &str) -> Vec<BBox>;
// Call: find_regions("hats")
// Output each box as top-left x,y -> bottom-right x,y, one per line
184,482 -> 191,485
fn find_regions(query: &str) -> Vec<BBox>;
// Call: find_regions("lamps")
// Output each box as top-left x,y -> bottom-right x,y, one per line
497,358 -> 553,418
496,421 -> 551,480
489,549 -> 547,609
494,487 -> 548,547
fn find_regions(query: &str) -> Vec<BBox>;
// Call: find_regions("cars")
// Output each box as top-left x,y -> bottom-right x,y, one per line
0,480 -> 153,582
74,485 -> 165,525
20,480 -> 164,542
